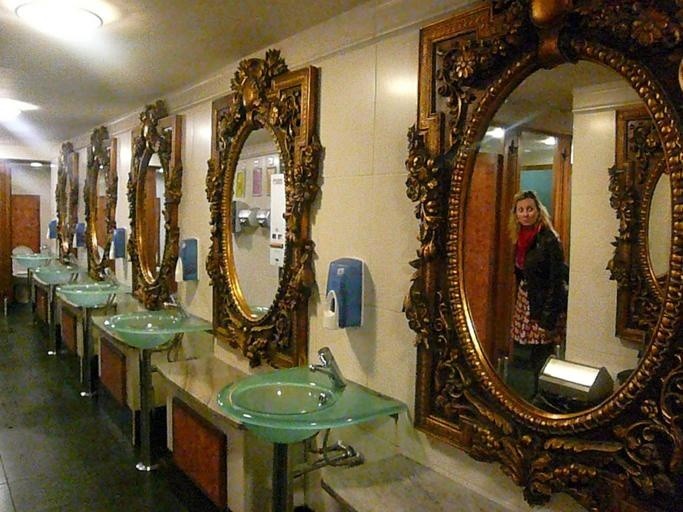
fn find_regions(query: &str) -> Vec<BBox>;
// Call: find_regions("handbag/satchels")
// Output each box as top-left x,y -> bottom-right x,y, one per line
558,262 -> 569,296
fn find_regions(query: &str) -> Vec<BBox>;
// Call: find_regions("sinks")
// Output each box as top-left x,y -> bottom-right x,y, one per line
10,254 -> 54,278
105,310 -> 213,350
56,282 -> 131,309
35,266 -> 99,284
218,364 -> 407,445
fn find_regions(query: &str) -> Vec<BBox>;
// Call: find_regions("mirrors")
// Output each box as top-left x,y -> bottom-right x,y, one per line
606,103 -> 674,345
204,49 -> 319,368
82,125 -> 118,282
402,2 -> 678,512
126,100 -> 182,310
56,142 -> 78,264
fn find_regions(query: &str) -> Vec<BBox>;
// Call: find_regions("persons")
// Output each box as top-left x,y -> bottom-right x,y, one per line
508,191 -> 569,403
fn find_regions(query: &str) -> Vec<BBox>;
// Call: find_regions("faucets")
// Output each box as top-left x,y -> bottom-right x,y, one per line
163,291 -> 191,319
62,252 -> 80,267
42,243 -> 51,256
99,266 -> 124,289
308,347 -> 348,391
